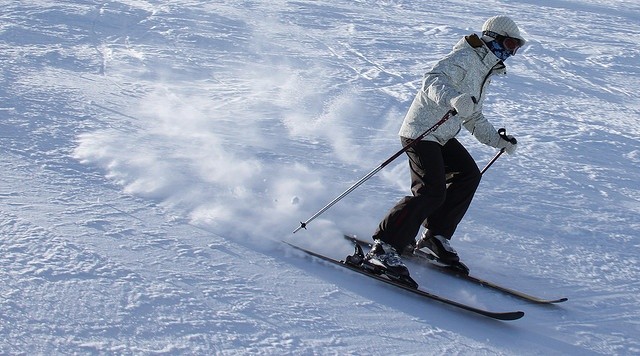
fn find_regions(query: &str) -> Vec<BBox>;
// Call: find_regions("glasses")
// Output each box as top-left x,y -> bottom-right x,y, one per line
482,31 -> 521,56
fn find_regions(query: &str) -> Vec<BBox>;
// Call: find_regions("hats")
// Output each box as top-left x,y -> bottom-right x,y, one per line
480,14 -> 526,46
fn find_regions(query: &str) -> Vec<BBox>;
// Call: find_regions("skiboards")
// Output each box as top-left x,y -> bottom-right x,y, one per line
282,233 -> 569,321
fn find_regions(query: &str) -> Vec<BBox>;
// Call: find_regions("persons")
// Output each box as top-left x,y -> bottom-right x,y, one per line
353,12 -> 528,281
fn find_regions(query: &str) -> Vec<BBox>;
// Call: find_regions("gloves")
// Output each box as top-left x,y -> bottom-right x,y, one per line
445,91 -> 474,118
496,135 -> 517,155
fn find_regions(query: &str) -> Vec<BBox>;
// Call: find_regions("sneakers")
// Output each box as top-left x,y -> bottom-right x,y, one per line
415,227 -> 459,262
364,239 -> 409,276
